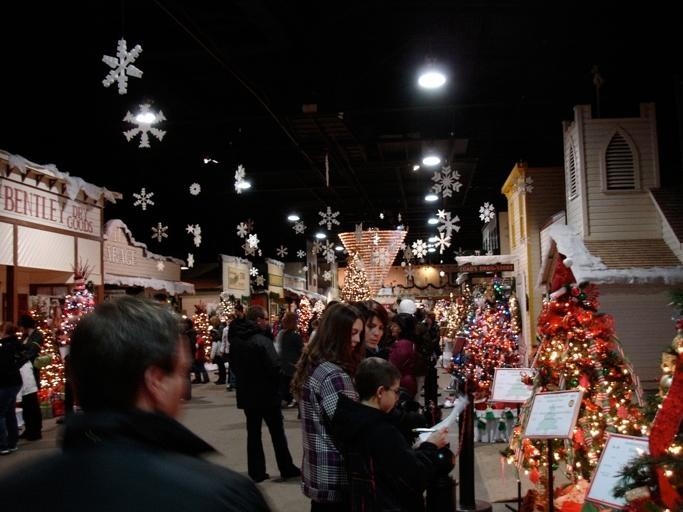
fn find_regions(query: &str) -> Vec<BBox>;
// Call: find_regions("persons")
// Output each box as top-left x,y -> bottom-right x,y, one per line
211,305 -> 245,390
285,299 -> 368,510
1,314 -> 43,453
346,299 -> 440,399
0,293 -> 273,512
56,358 -> 74,425
184,319 -> 209,400
229,305 -> 302,481
328,355 -> 450,510
278,312 -> 304,418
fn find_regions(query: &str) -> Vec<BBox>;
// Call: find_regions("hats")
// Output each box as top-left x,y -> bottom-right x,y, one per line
210,315 -> 221,325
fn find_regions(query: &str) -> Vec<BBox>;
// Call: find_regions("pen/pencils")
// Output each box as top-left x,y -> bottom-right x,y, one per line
412,427 -> 436,432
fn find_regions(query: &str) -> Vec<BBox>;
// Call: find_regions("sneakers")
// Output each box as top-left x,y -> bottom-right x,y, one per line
281,469 -> 302,482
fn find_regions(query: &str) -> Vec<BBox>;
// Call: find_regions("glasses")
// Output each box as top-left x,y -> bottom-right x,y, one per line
387,386 -> 400,395
263,317 -> 269,321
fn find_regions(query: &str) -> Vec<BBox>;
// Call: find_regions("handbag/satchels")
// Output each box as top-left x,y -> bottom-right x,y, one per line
30,342 -> 52,369
